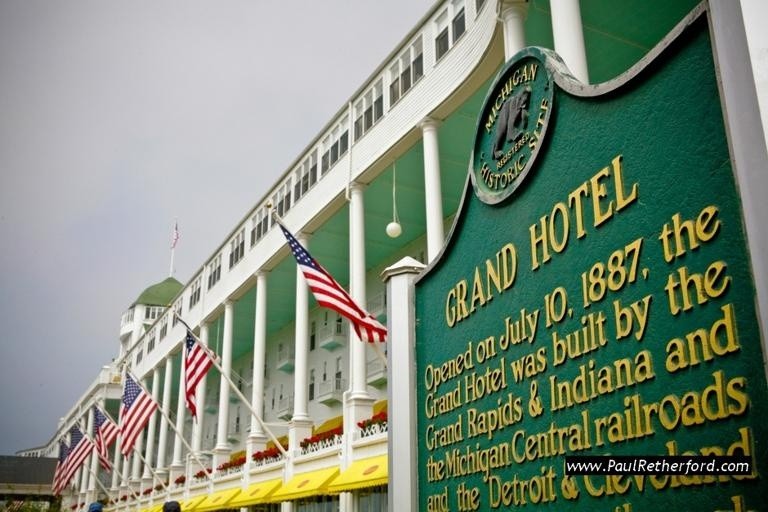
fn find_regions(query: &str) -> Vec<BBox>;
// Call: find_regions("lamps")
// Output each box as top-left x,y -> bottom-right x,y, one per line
386,156 -> 403,238
215,317 -> 221,364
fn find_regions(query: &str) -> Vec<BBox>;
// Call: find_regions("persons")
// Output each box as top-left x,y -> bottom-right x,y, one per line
89,502 -> 103,512
163,501 -> 181,512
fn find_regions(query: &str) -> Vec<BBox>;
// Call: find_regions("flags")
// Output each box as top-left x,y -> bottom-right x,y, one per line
120,372 -> 159,460
94,405 -> 120,470
53,423 -> 94,497
172,224 -> 180,247
280,221 -> 389,343
184,328 -> 214,423
